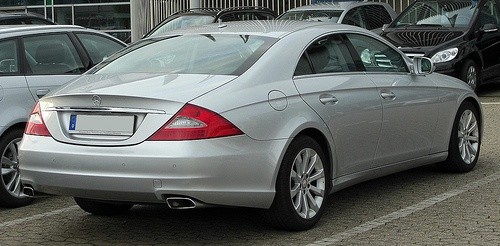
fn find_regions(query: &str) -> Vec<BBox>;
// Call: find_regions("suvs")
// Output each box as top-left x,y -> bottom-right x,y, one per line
379,1 -> 500,92
0,11 -> 54,27
140,5 -> 277,38
0,24 -> 130,210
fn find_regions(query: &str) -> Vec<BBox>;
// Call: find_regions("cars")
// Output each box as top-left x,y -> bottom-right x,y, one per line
273,2 -> 398,35
18,20 -> 483,229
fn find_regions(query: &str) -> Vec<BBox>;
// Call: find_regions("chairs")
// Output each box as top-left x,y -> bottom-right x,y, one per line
29,42 -> 71,75
296,44 -> 329,73
